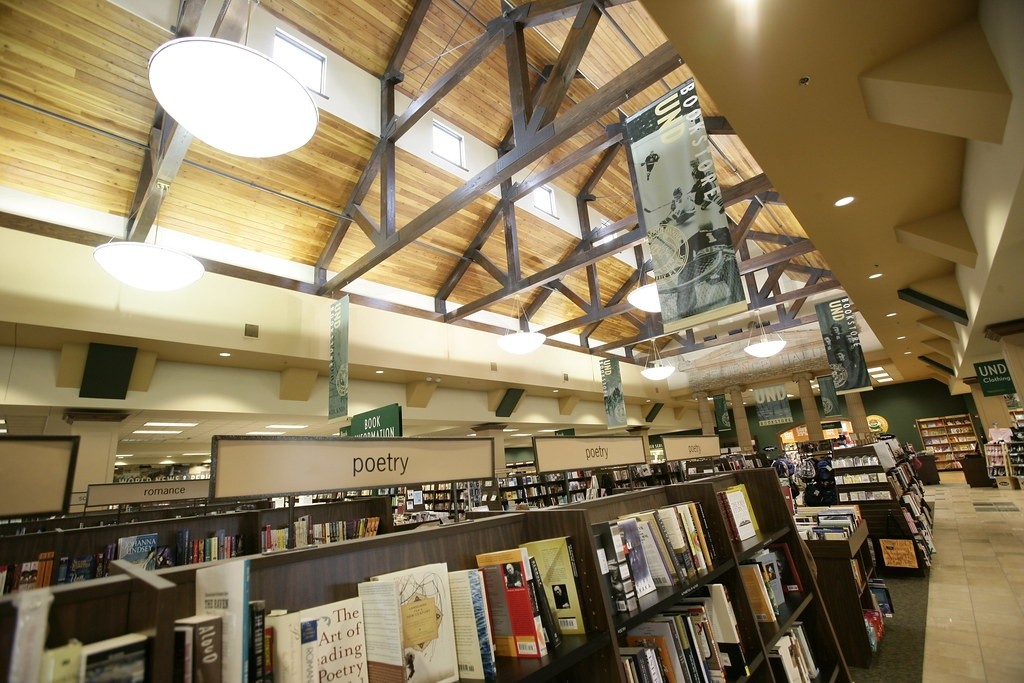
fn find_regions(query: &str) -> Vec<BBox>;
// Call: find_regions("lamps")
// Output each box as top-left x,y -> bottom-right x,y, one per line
641,339 -> 676,381
744,309 -> 786,357
145,0 -> 322,160
627,239 -> 661,313
498,294 -> 547,356
92,178 -> 205,293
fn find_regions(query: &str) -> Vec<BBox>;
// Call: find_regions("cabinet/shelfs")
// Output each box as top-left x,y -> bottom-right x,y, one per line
0,408 -> 1024,683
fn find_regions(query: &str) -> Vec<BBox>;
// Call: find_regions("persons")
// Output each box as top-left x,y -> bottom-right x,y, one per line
822,322 -> 860,370
553,586 -> 570,609
690,158 -> 725,214
641,151 -> 660,181
677,220 -> 745,313
158,547 -> 172,567
146,547 -> 156,560
506,564 -> 523,589
657,186 -> 696,229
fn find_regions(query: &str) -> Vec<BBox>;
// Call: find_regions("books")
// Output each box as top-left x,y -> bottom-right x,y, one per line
9,536 -> 592,683
379,471 -> 591,527
920,420 -> 976,468
794,505 -> 895,653
988,445 -> 1024,476
590,456 -> 821,683
261,514 -> 380,552
832,443 -> 937,567
0,529 -> 244,594
